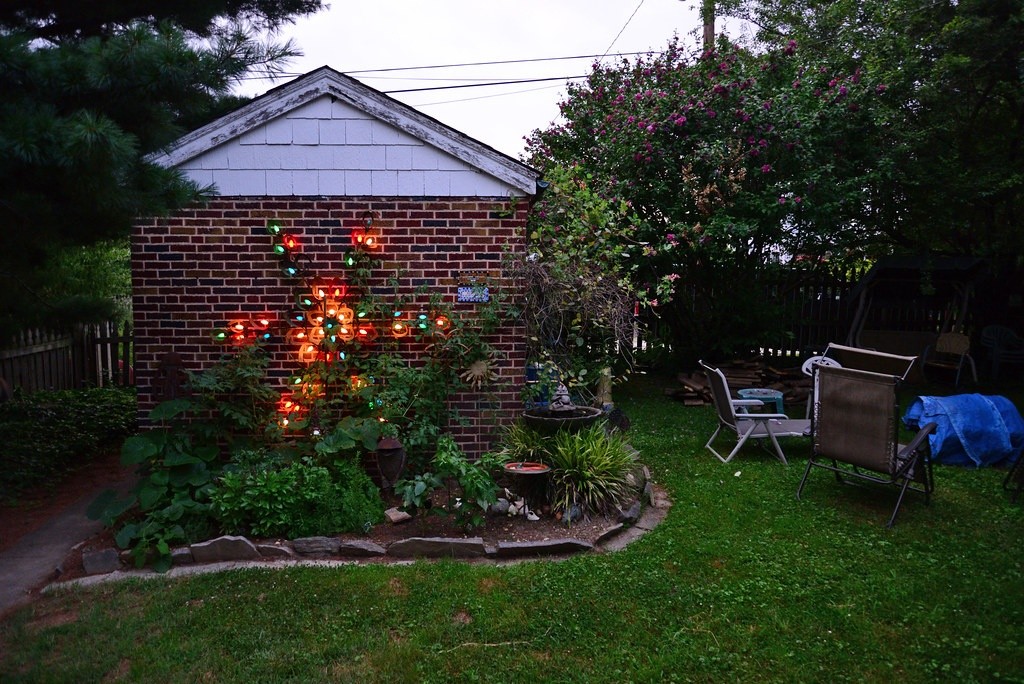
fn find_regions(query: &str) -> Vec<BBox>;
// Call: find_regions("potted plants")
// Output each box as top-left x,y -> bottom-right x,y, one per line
521,361 -> 610,433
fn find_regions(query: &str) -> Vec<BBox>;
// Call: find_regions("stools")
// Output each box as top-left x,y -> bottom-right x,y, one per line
738,388 -> 785,413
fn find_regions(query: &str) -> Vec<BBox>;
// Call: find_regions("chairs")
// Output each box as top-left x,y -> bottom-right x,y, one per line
802,356 -> 841,419
922,333 -> 978,386
698,360 -> 811,467
797,342 -> 938,530
